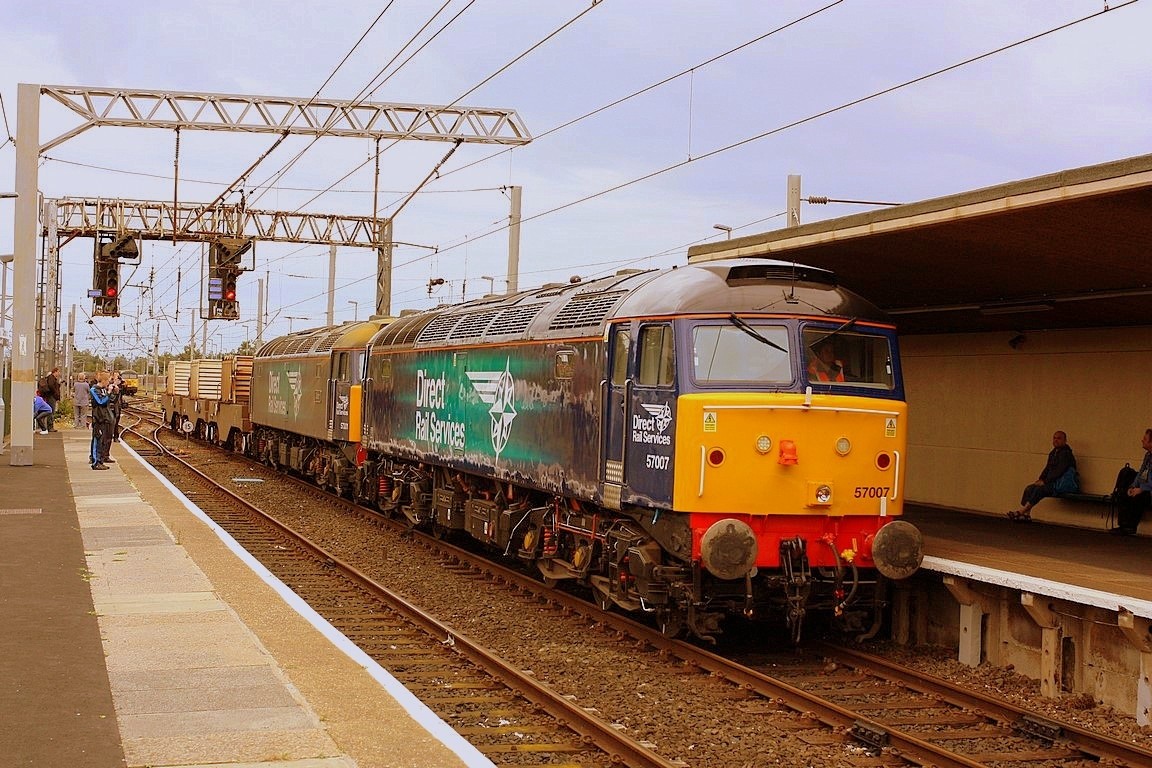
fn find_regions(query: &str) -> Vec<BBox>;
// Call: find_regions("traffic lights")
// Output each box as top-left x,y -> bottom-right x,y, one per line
225,272 -> 236,301
106,268 -> 118,297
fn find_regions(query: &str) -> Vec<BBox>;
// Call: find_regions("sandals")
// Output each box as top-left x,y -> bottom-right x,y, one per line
1006,509 -> 1033,522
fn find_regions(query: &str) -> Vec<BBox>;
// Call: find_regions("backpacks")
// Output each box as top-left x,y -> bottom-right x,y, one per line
38,377 -> 50,396
1114,462 -> 1138,496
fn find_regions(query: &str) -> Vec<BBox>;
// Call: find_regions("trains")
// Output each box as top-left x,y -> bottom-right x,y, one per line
64,368 -> 139,397
154,253 -> 927,661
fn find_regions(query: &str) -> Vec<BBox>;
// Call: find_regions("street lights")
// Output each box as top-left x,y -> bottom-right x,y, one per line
347,300 -> 358,322
712,224 -> 733,241
242,324 -> 249,356
209,338 -> 213,358
480,275 -> 494,295
216,333 -> 222,359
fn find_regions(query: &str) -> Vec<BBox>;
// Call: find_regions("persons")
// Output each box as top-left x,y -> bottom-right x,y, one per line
1109,428 -> 1152,534
34,368 -> 128,470
807,342 -> 845,382
1006,431 -> 1081,523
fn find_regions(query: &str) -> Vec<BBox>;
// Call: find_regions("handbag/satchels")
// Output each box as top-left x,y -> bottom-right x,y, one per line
1053,468 -> 1081,494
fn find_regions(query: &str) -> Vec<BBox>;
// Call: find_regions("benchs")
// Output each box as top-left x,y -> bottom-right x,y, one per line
1056,494 -> 1112,503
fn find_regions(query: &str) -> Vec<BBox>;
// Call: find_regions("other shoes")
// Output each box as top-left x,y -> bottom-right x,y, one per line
88,456 -> 115,463
39,430 -> 48,435
1109,527 -> 1137,535
49,428 -> 58,432
92,464 -> 110,470
114,438 -> 120,443
